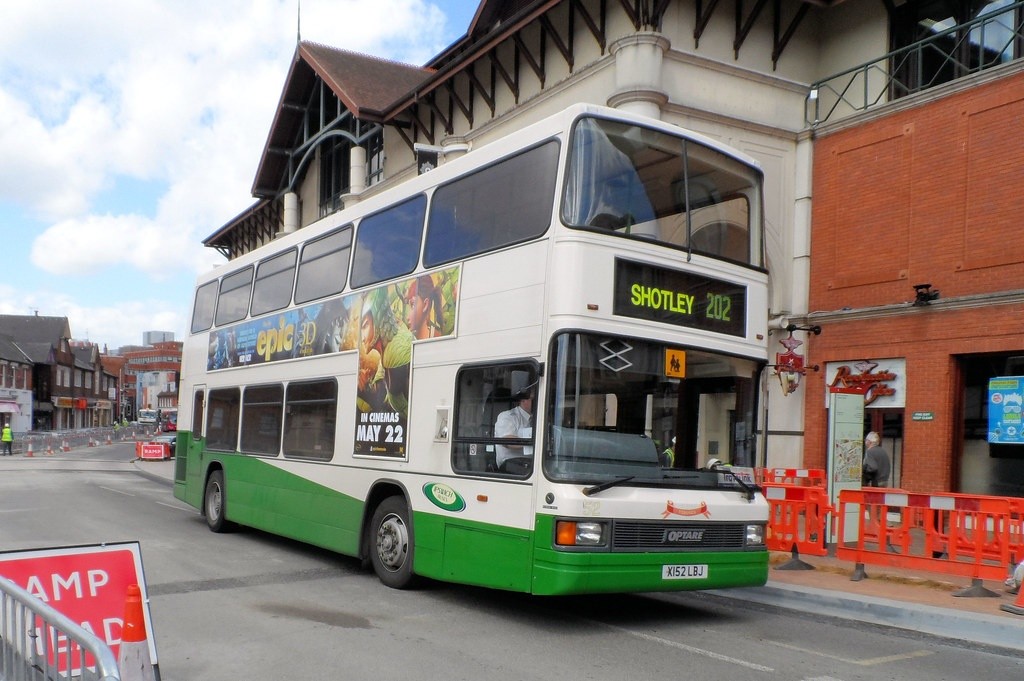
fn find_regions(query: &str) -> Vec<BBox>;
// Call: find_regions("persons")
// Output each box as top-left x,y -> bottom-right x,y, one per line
707,458 -> 732,470
122,418 -> 128,427
861,431 -> 891,524
494,388 -> 534,474
663,436 -> 676,469
114,421 -> 120,432
1,423 -> 14,456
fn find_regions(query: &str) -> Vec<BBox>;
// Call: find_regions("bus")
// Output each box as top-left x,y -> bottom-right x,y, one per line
172,102 -> 798,596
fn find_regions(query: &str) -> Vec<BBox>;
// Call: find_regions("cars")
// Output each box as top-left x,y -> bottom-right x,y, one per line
151,436 -> 176,460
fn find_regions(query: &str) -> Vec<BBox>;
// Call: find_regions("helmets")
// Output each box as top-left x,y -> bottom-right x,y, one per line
707,458 -> 722,469
671,437 -> 675,444
4,423 -> 10,428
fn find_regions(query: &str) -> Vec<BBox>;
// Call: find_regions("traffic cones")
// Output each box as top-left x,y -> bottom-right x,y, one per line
89,435 -> 93,446
27,440 -> 33,457
47,438 -> 54,456
62,438 -> 71,452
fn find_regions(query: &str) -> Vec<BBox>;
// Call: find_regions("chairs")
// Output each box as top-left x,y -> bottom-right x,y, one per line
483,387 -> 512,474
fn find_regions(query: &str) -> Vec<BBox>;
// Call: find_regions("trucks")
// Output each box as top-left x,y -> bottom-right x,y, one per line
157,407 -> 177,432
138,408 -> 160,432
